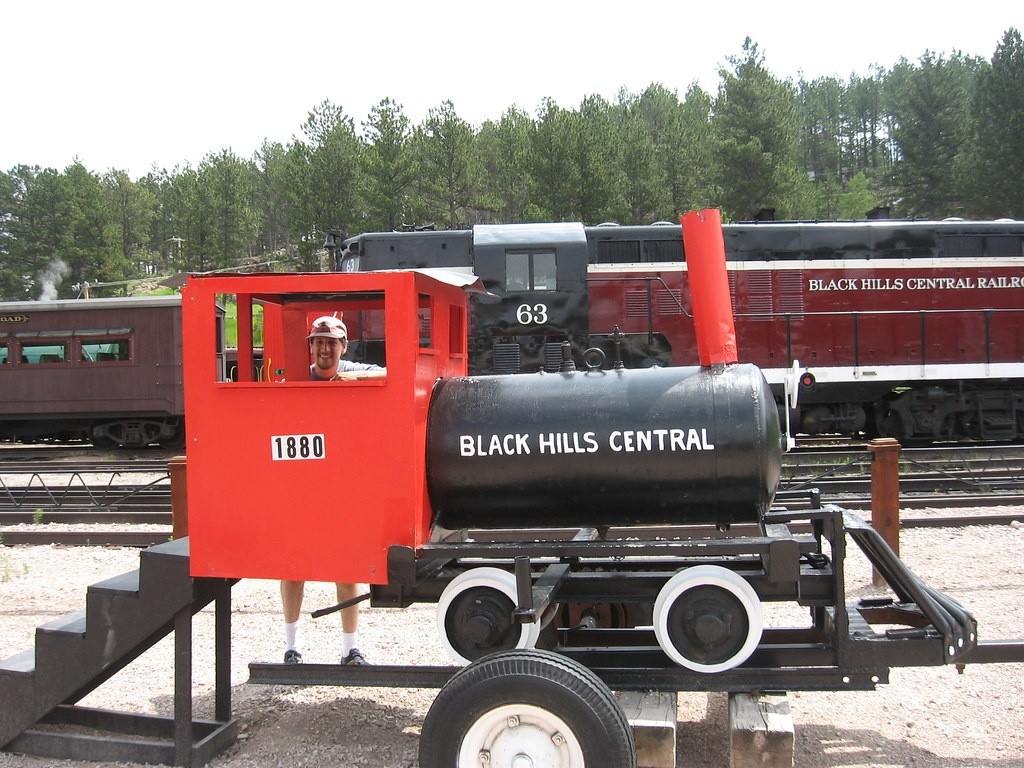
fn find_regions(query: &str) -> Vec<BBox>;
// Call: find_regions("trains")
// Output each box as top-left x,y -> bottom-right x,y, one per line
322,218 -> 1023,449
0,297 -> 227,449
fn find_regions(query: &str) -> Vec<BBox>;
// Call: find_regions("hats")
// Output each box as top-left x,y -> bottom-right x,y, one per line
306,316 -> 347,339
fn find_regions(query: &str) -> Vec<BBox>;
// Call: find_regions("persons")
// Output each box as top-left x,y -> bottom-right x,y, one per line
278,316 -> 387,665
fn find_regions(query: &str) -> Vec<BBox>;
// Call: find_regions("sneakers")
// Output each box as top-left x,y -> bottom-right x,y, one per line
341,649 -> 371,665
284,650 -> 303,664
